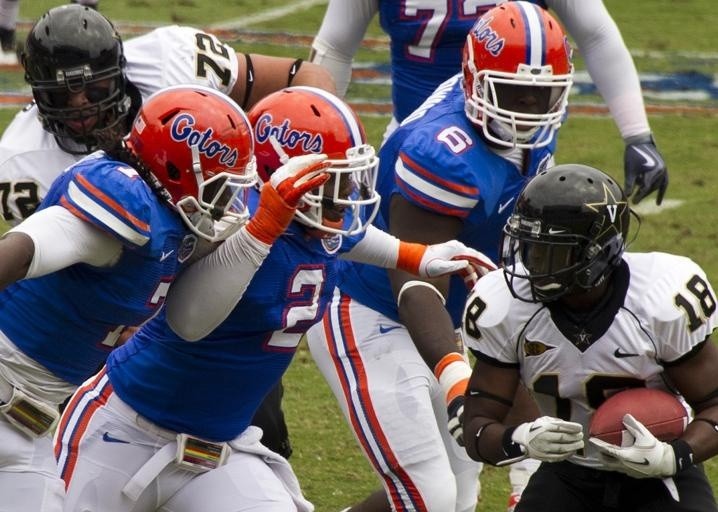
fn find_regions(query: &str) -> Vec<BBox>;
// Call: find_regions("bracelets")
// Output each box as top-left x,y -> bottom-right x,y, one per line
502,426 -> 521,458
672,439 -> 695,475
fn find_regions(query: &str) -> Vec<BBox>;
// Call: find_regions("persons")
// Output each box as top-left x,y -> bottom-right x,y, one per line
457,164 -> 717,512
0,4 -> 337,461
52,86 -> 500,511
0,83 -> 259,512
0,0 -> 99,67
309,0 -> 575,512
310,0 -> 667,512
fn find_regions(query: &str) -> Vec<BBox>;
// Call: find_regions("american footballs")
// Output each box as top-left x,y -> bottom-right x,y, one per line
588,388 -> 687,456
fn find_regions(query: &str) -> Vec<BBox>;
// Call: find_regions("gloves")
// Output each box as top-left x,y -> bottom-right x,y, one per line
431,352 -> 473,446
494,415 -> 585,468
397,233 -> 498,289
247,153 -> 332,244
589,412 -> 695,480
621,130 -> 669,205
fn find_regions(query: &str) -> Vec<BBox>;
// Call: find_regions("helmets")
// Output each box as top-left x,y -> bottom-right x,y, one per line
19,2 -> 127,154
498,164 -> 641,304
130,84 -> 259,244
462,0 -> 576,157
249,85 -> 382,237
